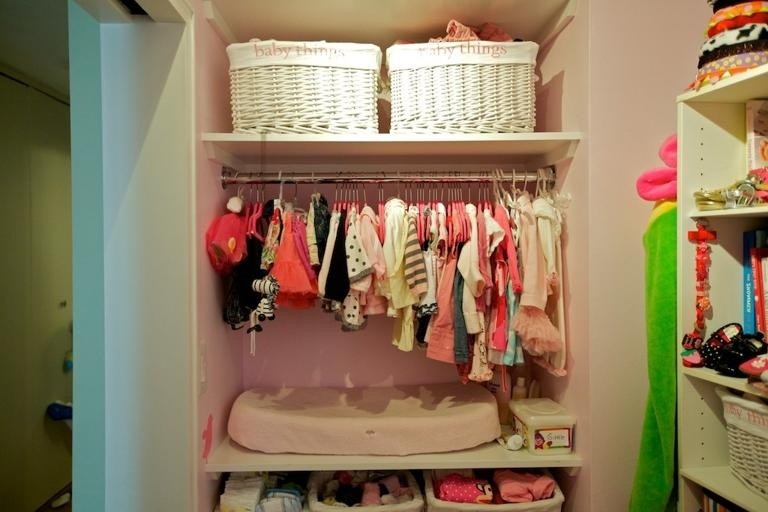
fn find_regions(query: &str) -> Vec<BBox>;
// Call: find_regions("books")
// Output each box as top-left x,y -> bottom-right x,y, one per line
743,96 -> 768,175
743,228 -> 768,343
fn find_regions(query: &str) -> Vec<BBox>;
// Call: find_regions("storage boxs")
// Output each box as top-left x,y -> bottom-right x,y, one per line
509,399 -> 578,457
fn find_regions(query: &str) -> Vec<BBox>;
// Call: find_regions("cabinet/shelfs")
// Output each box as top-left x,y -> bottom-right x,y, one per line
203,116 -> 582,510
676,64 -> 767,510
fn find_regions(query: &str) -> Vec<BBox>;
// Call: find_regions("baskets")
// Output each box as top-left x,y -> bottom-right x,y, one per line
224,37 -> 383,134
382,38 -> 540,135
709,385 -> 768,502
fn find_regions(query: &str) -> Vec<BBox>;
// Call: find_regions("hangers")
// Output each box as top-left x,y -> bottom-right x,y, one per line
234,167 -> 554,245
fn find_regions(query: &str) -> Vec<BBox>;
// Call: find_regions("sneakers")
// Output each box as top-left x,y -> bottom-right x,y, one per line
699,322 -> 768,383
